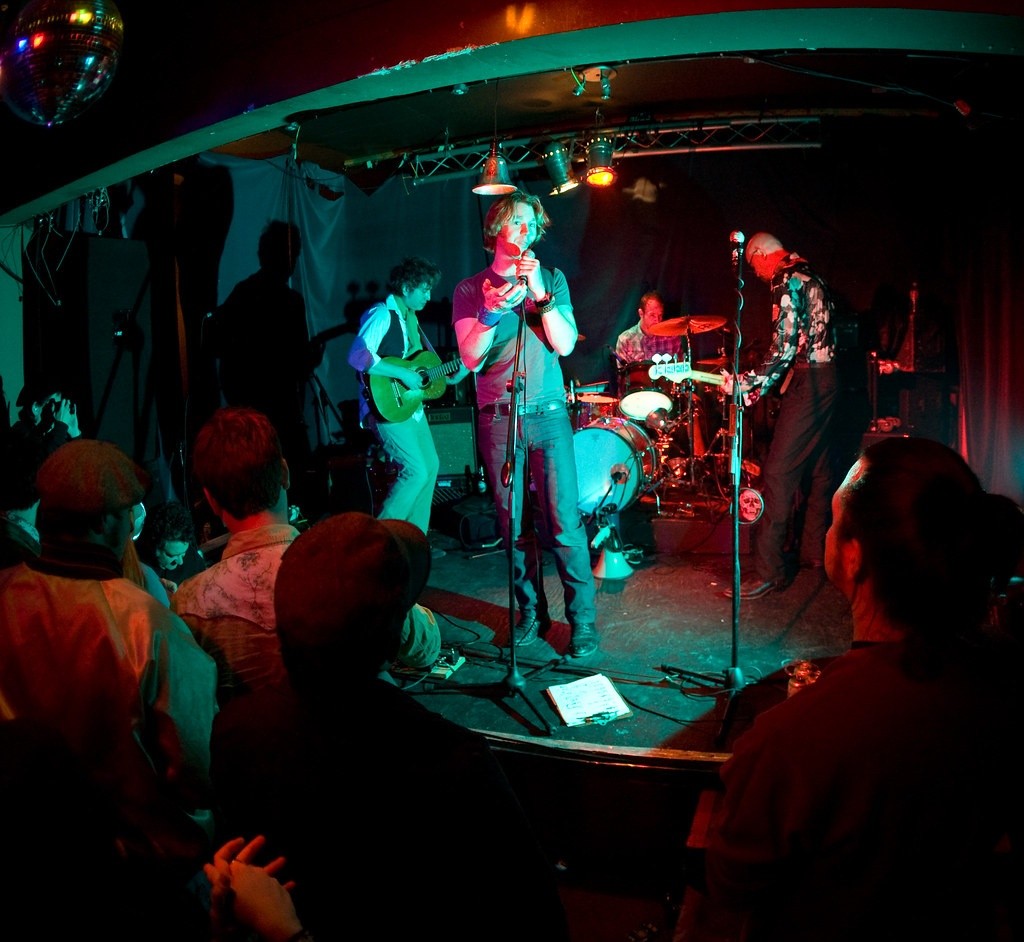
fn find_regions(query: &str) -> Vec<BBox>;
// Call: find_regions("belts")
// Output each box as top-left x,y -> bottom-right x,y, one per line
779,364 -> 835,395
480,400 -> 564,417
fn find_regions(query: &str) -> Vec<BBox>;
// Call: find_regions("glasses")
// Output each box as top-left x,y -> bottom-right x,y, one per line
745,251 -> 758,272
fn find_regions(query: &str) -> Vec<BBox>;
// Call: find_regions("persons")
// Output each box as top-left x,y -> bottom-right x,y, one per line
171,410 -> 443,708
704,436 -> 1024,942
0,439 -> 223,860
0,718 -> 314,942
348,261 -> 472,536
148,506 -> 199,585
615,294 -> 686,473
453,190 -> 600,656
724,235 -> 836,601
210,512 -> 570,942
0,378 -> 85,513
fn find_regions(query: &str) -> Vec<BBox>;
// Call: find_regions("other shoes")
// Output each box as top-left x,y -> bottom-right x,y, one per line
627,553 -> 655,564
430,546 -> 446,559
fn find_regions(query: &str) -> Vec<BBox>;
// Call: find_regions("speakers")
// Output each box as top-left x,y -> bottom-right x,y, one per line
423,406 -> 479,477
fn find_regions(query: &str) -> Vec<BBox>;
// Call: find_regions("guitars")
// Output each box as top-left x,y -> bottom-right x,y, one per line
645,352 -> 769,398
365,350 -> 462,424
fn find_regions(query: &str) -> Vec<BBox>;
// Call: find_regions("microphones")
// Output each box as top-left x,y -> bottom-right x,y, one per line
607,345 -> 628,367
518,249 -> 535,284
729,230 -> 745,272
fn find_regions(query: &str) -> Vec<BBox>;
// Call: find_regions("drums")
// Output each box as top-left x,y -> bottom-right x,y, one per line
572,392 -> 619,429
571,415 -> 656,513
618,359 -> 676,423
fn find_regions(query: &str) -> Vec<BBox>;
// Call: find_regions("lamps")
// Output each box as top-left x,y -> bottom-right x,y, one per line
542,142 -> 580,196
571,63 -> 616,100
587,134 -> 618,189
471,78 -> 517,195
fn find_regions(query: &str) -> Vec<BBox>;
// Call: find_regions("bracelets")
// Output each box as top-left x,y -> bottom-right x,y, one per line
478,305 -> 503,327
538,304 -> 554,314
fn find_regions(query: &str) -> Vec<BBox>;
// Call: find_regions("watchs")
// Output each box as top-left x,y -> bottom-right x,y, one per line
534,291 -> 556,308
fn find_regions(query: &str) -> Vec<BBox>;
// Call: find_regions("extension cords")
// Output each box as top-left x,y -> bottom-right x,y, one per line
428,655 -> 466,679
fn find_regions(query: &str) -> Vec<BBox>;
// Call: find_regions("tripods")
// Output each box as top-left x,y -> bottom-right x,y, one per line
423,285 -> 571,735
660,274 -> 791,748
643,327 -> 732,524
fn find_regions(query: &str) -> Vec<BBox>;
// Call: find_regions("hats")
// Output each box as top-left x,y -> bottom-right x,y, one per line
35,439 -> 150,514
274,511 -> 430,655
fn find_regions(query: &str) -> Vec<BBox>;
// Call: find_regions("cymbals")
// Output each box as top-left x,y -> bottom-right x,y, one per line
647,314 -> 729,337
696,357 -> 726,365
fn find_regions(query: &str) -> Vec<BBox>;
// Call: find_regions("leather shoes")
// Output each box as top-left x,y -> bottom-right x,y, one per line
513,612 -> 542,646
571,623 -> 598,656
784,552 -> 824,568
724,573 -> 787,600
